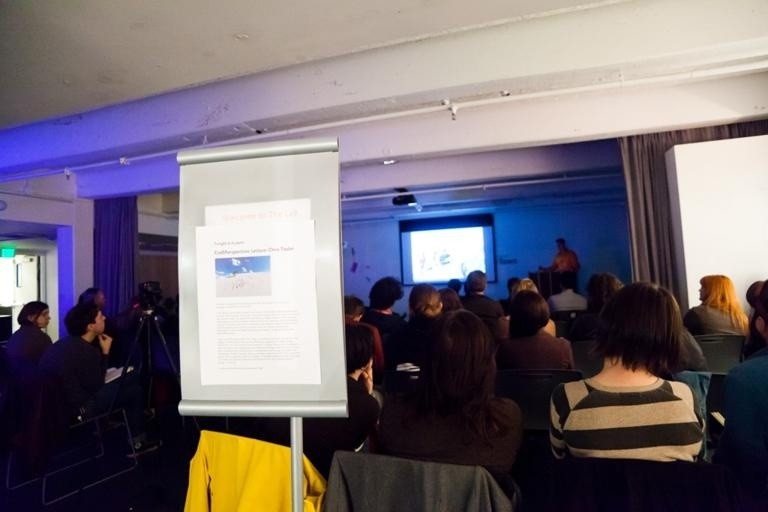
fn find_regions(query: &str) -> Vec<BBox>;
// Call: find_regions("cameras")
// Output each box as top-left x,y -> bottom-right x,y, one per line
138,281 -> 163,311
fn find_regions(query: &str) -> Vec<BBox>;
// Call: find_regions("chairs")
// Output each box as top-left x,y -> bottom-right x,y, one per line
184,429 -> 325,511
0,311 -> 177,511
343,309 -> 745,458
322,453 -> 515,510
558,457 -> 717,509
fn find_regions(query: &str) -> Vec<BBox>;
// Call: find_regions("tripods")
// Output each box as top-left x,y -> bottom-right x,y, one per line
88,310 -> 201,463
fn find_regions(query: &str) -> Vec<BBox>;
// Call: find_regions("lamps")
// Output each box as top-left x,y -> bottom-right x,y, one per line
0,243 -> 17,258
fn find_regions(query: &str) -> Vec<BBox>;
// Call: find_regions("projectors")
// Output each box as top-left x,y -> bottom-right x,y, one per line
392,188 -> 418,207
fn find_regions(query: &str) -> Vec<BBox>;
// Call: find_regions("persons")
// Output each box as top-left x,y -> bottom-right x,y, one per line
302,269 -> 768,511
1,287 -> 265,512
542,239 -> 580,272
